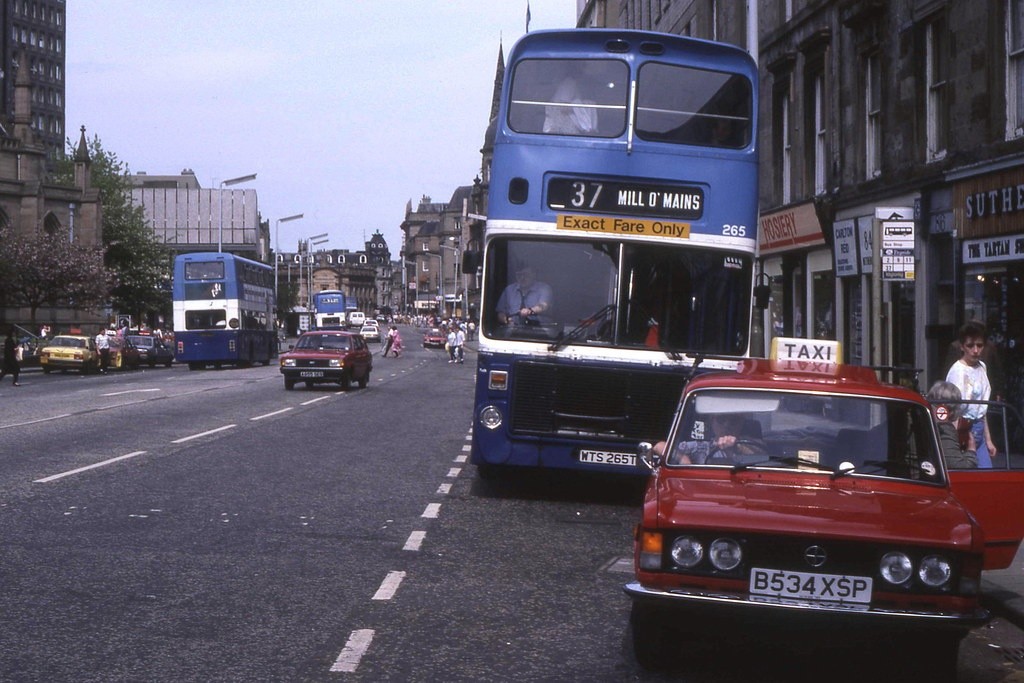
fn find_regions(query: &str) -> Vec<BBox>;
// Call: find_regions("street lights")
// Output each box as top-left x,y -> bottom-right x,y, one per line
405,259 -> 418,316
425,253 -> 442,316
306,232 -> 330,309
439,245 -> 459,318
275,213 -> 304,300
218,173 -> 258,254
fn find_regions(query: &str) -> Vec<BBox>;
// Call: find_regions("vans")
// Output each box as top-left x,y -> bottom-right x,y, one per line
347,311 -> 366,328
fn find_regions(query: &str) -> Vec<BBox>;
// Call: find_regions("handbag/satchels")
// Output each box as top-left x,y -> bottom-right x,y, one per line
16,347 -> 24,360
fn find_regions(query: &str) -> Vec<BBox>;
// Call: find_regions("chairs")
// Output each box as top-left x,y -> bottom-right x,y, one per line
696,415 -> 763,443
825,430 -> 896,467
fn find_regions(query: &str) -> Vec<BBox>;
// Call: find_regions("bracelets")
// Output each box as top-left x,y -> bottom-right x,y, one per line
529,307 -> 534,314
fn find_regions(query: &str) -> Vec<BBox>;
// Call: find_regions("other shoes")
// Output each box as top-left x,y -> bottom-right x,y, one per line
13,382 -> 19,386
100,369 -> 102,372
104,372 -> 107,375
448,358 -> 464,363
397,355 -> 402,358
381,355 -> 385,358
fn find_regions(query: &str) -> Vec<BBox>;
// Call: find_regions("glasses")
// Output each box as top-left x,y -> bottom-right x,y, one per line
711,414 -> 741,424
516,271 -> 533,278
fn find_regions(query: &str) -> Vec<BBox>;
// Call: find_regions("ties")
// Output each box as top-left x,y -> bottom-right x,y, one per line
519,289 -> 531,309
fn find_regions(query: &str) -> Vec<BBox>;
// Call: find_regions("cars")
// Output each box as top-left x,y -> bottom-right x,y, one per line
359,318 -> 382,342
92,336 -> 140,369
127,335 -> 174,369
621,359 -> 1024,683
39,335 -> 99,373
422,327 -> 448,348
280,331 -> 374,392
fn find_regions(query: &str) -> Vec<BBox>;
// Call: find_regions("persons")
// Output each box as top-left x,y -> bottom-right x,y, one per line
494,262 -> 555,325
946,321 -> 997,468
653,413 -> 767,465
887,382 -> 977,480
447,328 -> 457,363
381,326 -> 393,357
0,329 -> 20,386
388,313 -> 476,341
390,326 -> 400,358
95,328 -> 120,375
41,324 -> 50,337
454,326 -> 465,362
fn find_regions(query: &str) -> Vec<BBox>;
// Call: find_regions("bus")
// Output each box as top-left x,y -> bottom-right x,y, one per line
314,290 -> 348,331
460,29 -> 775,481
173,252 -> 279,370
